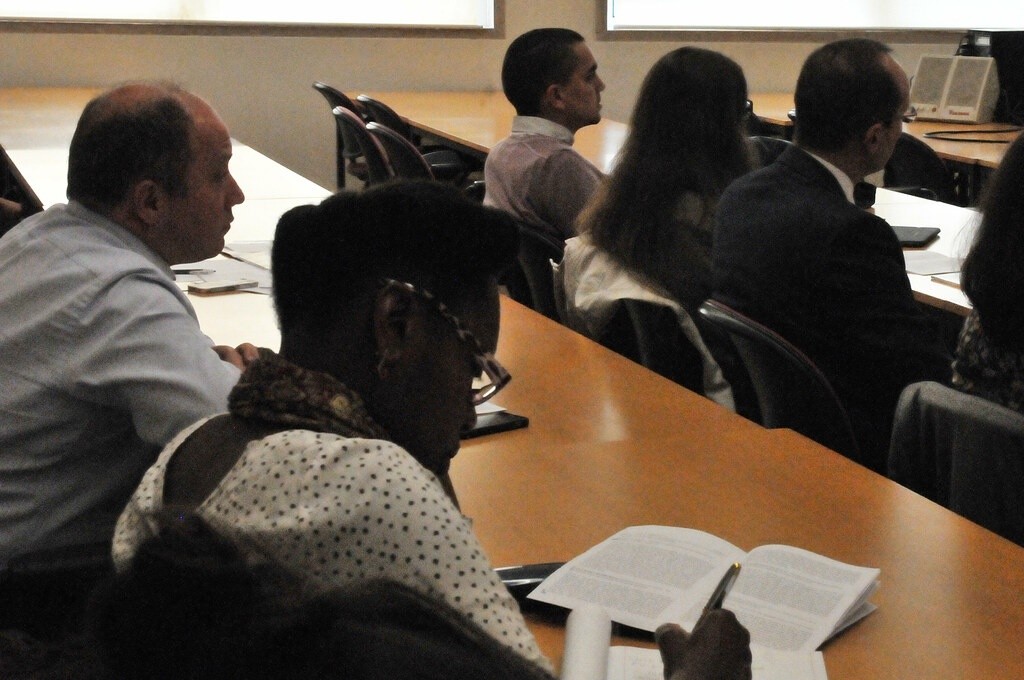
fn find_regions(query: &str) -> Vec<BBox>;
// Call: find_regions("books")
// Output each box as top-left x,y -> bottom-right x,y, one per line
527,525 -> 881,653
561,606 -> 827,680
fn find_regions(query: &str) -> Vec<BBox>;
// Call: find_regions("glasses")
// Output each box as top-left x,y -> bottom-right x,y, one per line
737,100 -> 755,122
370,279 -> 512,404
891,105 -> 917,124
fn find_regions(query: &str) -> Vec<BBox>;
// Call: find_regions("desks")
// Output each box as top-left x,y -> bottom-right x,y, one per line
442,423 -> 1023,680
1,82 -> 769,537
344,86 -> 982,322
747,92 -> 1023,199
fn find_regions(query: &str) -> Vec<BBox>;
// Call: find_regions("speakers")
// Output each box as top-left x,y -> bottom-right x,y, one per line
908,52 -> 1000,126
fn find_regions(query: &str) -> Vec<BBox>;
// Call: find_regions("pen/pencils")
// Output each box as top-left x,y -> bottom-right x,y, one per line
693,561 -> 742,638
171,269 -> 217,274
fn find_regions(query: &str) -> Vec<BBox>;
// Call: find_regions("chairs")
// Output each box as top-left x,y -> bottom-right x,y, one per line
317,83 -> 1024,542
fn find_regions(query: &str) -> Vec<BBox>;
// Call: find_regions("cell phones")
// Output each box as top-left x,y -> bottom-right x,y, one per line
460,412 -> 531,439
187,276 -> 259,294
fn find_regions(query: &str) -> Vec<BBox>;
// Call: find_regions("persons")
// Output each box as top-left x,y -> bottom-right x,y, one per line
946,132 -> 1024,408
0,85 -> 261,609
483,28 -> 606,246
714,40 -> 945,460
113,180 -> 753,680
576,46 -> 752,409
